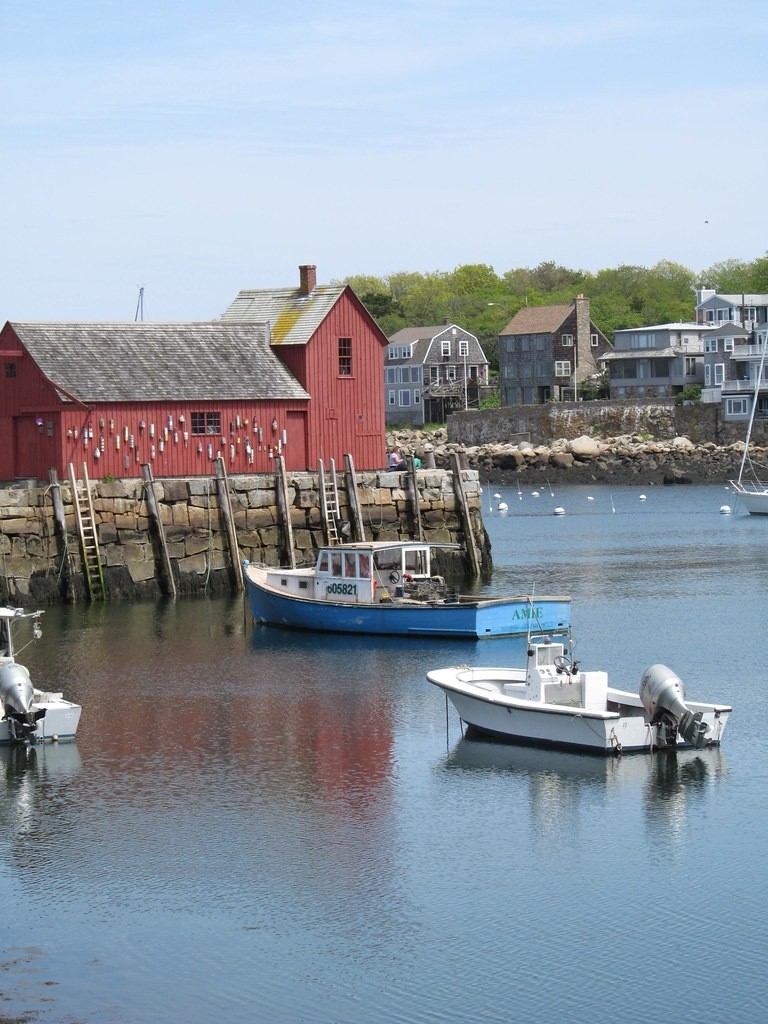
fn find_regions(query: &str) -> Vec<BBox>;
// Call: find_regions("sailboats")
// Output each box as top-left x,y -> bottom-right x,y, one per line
726,328 -> 768,517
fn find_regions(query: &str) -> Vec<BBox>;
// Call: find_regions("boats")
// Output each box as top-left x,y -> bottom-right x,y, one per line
440,723 -> 723,845
1,601 -> 83,744
241,540 -> 575,641
426,584 -> 732,754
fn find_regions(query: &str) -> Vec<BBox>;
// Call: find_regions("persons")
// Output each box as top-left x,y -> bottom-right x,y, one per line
391,446 -> 406,470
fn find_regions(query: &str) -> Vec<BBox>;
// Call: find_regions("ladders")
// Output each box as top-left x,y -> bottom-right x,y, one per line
67,461 -> 107,603
318,458 -> 343,546
